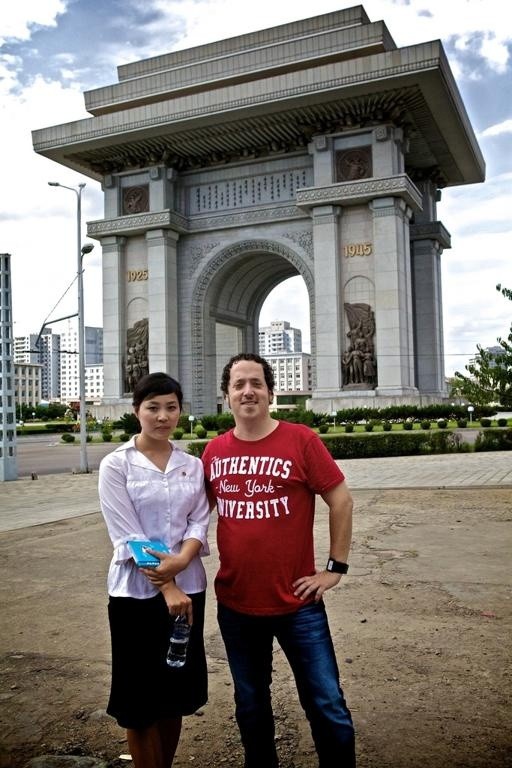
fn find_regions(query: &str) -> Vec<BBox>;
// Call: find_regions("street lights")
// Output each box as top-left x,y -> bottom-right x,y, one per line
32,412 -> 36,423
188,414 -> 195,437
467,405 -> 474,426
330,410 -> 338,432
48,180 -> 93,475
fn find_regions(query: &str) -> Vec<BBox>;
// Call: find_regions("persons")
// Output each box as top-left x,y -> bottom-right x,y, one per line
341,316 -> 377,389
95,372 -> 214,767
122,332 -> 150,393
199,353 -> 357,767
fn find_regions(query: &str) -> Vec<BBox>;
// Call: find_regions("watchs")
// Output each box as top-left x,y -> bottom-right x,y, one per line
325,557 -> 350,575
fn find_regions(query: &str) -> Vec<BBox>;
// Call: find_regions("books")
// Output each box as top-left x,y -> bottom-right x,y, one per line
127,537 -> 170,568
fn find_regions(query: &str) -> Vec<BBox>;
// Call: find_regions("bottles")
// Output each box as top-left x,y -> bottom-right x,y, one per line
164,609 -> 193,668
